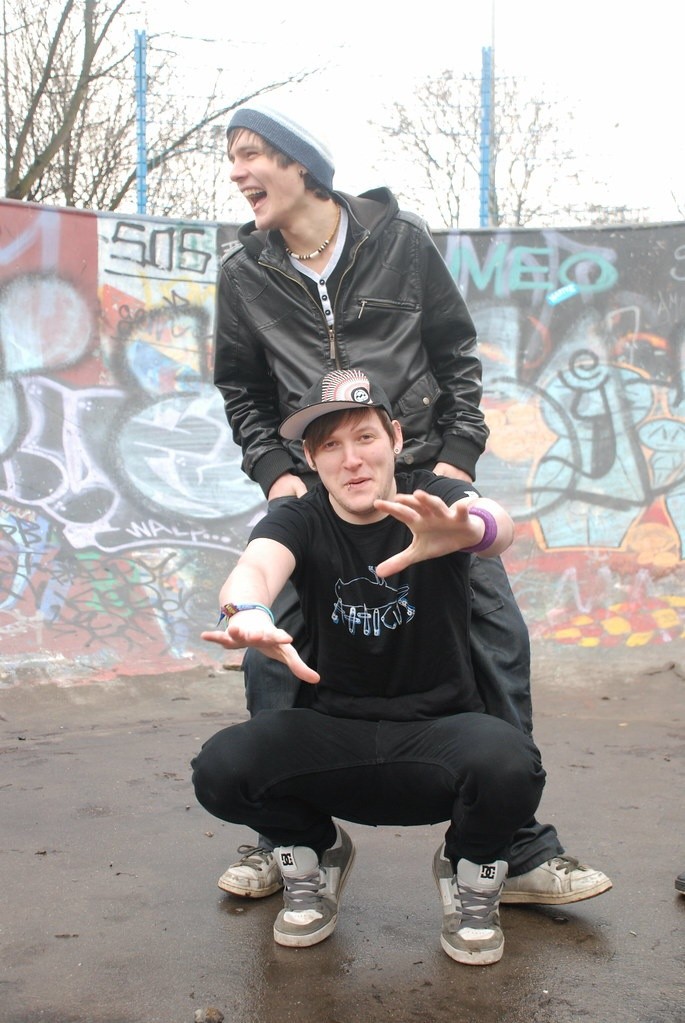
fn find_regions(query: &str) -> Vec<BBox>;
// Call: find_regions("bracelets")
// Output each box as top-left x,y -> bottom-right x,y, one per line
461,506 -> 497,553
217,602 -> 274,630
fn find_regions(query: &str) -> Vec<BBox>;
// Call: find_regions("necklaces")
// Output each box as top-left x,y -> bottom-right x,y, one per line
282,200 -> 342,260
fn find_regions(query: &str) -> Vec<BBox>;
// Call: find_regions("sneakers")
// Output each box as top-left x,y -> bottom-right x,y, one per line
498,852 -> 612,904
433,840 -> 509,964
274,821 -> 357,948
217,843 -> 284,897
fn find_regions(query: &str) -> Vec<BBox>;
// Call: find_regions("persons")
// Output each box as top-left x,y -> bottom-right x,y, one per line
192,367 -> 547,970
209,92 -> 614,907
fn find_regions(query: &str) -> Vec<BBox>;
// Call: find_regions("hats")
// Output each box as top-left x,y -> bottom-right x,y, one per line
279,369 -> 393,442
226,81 -> 335,192
